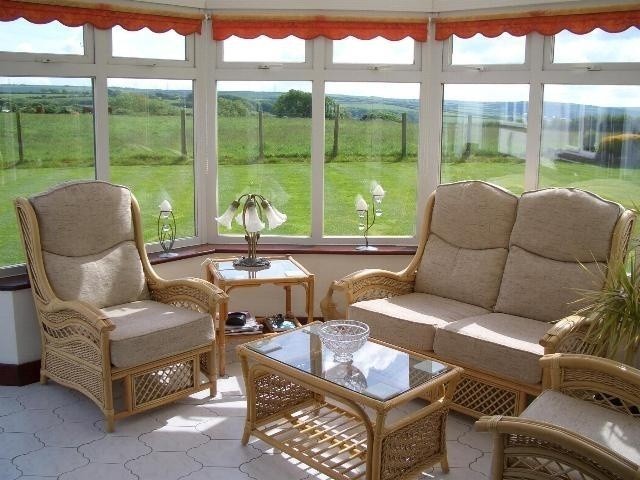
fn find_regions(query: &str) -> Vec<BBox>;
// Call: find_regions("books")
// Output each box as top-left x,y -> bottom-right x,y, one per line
214,309 -> 297,336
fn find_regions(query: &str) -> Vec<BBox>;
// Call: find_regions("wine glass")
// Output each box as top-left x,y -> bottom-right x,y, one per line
317,320 -> 370,362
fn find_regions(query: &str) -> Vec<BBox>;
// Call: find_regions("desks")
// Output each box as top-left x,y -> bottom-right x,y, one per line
206,253 -> 315,377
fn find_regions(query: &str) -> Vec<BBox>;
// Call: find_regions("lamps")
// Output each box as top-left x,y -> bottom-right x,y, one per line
213,194 -> 288,266
157,198 -> 177,258
355,184 -> 388,251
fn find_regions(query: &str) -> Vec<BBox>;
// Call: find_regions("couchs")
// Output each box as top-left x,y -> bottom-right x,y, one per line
321,179 -> 637,419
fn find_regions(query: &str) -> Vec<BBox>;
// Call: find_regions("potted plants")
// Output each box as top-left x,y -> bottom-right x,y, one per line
563,195 -> 640,415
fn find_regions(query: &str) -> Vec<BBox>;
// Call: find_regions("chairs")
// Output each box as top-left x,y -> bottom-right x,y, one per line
473,351 -> 639,480
12,179 -> 230,432
